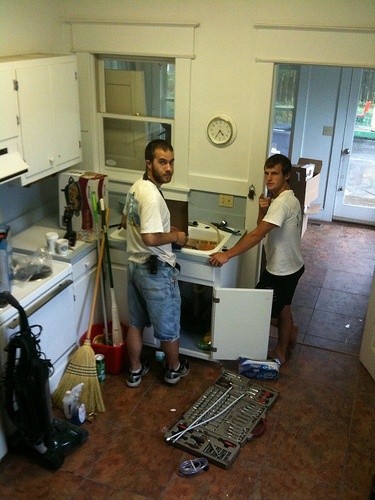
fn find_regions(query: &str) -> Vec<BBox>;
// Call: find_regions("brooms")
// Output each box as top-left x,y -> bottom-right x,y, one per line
51,234 -> 106,413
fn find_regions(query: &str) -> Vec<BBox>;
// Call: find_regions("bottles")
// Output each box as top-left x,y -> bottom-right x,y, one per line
71,393 -> 87,426
62,390 -> 74,419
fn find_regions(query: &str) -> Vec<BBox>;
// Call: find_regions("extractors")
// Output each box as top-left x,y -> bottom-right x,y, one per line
0,140 -> 28,186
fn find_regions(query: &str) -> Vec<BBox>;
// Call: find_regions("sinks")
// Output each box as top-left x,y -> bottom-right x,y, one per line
109,226 -> 128,241
180,223 -> 234,258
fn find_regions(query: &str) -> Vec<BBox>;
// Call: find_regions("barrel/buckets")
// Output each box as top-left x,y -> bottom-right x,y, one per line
80,323 -> 128,375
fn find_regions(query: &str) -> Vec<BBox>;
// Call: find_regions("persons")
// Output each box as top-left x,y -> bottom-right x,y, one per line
120,139 -> 191,387
209,154 -> 305,366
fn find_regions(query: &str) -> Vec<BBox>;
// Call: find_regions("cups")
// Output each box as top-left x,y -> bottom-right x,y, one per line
45,232 -> 59,253
56,239 -> 69,255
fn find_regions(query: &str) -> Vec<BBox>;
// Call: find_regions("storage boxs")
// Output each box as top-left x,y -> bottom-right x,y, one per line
58,170 -> 110,234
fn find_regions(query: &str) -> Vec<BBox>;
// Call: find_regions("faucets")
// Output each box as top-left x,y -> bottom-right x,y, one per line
199,221 -> 221,244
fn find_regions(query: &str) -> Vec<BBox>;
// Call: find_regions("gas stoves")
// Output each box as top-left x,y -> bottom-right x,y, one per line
0,253 -> 73,322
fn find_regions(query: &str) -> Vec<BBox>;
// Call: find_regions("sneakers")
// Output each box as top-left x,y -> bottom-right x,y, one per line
125,360 -> 150,388
163,358 -> 191,384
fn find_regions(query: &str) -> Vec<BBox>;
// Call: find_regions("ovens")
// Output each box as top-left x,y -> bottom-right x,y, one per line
0,273 -> 77,411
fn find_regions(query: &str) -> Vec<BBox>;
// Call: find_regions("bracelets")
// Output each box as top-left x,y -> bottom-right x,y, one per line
175,231 -> 179,243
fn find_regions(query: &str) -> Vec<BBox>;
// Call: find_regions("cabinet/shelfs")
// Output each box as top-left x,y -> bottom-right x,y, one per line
0,52 -> 84,188
105,247 -> 274,362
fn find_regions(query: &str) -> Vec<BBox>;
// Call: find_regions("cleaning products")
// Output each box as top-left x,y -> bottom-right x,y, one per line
62,382 -> 88,426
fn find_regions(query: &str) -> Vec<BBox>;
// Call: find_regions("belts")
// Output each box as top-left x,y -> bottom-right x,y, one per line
143,259 -> 182,275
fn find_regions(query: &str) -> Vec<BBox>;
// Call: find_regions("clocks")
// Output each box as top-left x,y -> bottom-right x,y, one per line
205,115 -> 238,147
